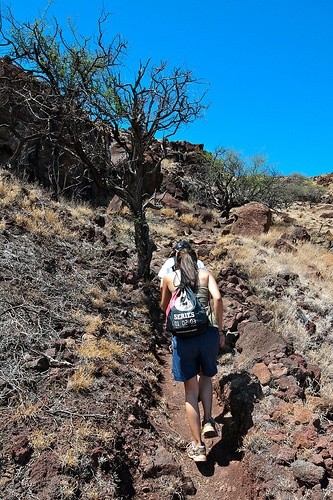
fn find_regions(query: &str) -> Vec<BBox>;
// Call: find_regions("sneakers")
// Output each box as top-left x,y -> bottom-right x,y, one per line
202,420 -> 219,439
187,441 -> 208,462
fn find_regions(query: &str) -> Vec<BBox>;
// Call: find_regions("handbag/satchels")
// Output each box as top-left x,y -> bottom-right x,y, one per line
165,272 -> 210,337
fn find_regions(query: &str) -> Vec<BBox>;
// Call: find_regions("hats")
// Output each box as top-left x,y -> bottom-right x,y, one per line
167,241 -> 190,257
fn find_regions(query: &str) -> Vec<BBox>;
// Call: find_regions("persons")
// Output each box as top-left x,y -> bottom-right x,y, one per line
158,241 -> 206,352
161,249 -> 226,462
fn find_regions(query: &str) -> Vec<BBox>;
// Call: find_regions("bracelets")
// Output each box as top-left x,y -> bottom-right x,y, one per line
219,334 -> 223,336
218,330 -> 223,333
